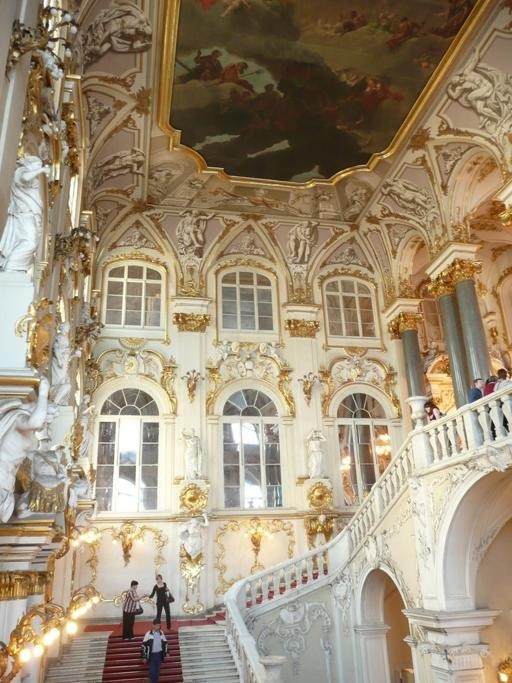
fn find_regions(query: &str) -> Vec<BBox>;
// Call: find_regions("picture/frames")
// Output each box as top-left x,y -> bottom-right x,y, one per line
156,1 -> 503,192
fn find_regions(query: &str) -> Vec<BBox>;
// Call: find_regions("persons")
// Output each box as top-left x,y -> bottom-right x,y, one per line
89,99 -> 111,124
87,5 -> 152,55
4,155 -> 51,272
296,220 -> 319,259
132,227 -> 145,246
149,169 -> 171,196
424,402 -> 444,425
446,47 -> 501,126
75,394 -> 96,458
93,148 -> 145,188
141,619 -> 168,683
246,238 -> 264,255
180,426 -> 202,480
146,574 -> 175,631
382,179 -> 431,210
306,430 -> 327,477
177,513 -> 210,558
0,376 -> 60,525
468,368 -> 512,439
121,580 -> 146,641
179,208 -> 215,248
51,320 -> 71,384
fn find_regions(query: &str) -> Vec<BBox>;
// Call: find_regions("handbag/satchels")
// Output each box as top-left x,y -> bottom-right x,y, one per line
134,608 -> 143,615
166,590 -> 174,603
